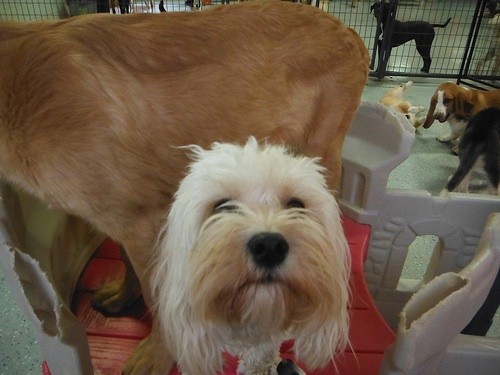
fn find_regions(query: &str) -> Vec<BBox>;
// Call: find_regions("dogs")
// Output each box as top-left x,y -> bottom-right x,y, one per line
0,0 -> 372,320
379,80 -> 427,129
422,81 -> 500,193
369,0 -> 453,79
148,134 -> 352,374
